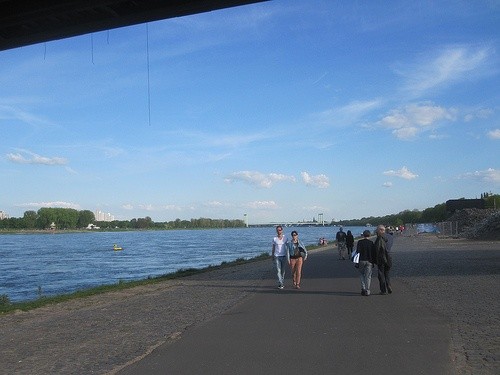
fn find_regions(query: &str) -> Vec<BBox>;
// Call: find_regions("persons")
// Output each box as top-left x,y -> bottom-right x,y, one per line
335,227 -> 347,261
318,237 -> 328,246
272,226 -> 288,290
287,231 -> 308,289
385,225 -> 405,235
354,230 -> 374,296
346,229 -> 355,261
373,224 -> 393,295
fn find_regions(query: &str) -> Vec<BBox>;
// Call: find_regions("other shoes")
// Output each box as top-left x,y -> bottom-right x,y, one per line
361,288 -> 370,296
381,287 -> 392,295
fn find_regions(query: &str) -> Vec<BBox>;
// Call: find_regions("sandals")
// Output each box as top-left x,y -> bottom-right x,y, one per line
293,281 -> 301,289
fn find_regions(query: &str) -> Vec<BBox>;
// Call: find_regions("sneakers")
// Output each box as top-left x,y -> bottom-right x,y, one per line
278,283 -> 284,289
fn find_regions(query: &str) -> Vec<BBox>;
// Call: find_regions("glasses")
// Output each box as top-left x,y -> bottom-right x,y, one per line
277,230 -> 282,231
292,234 -> 297,236
